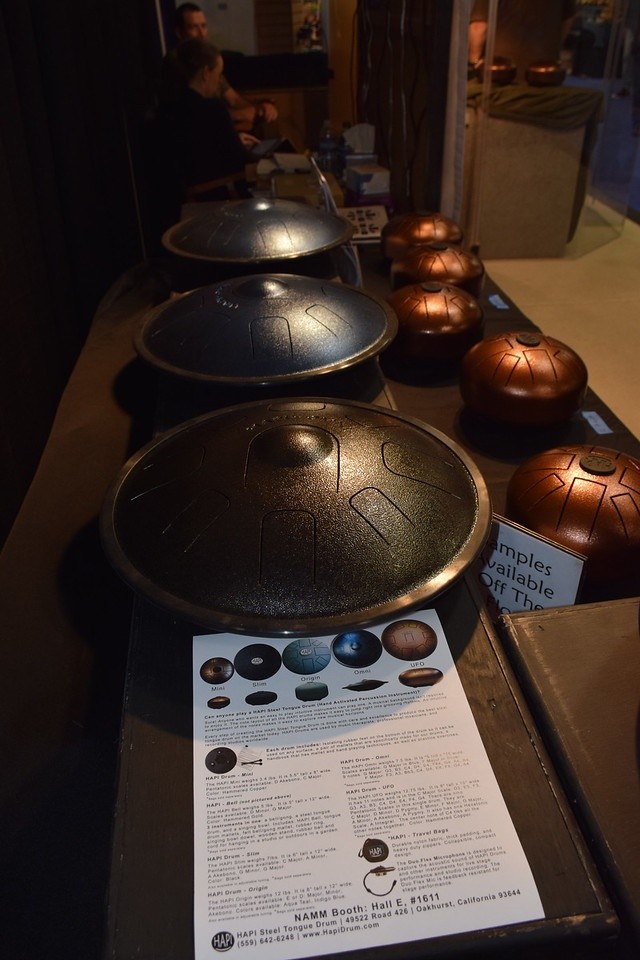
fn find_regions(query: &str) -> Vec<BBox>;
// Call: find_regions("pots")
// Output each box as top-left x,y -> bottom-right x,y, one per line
133,273 -> 398,390
388,281 -> 485,372
489,57 -> 517,85
526,61 -> 565,87
378,210 -> 463,259
392,242 -> 485,297
97,396 -> 493,639
161,197 -> 355,274
458,331 -> 586,433
200,619 -> 443,710
501,445 -> 640,599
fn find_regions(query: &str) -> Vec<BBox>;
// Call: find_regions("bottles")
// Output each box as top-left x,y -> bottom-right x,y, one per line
335,122 -> 351,177
318,120 -> 338,172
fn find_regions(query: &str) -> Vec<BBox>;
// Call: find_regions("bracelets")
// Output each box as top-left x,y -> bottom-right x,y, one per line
254,104 -> 265,118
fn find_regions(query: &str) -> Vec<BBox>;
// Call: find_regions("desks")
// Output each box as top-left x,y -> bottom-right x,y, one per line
0,174 -> 640,960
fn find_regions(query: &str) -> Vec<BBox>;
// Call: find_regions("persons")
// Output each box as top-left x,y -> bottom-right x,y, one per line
139,2 -> 277,236
556,35 -> 592,90
